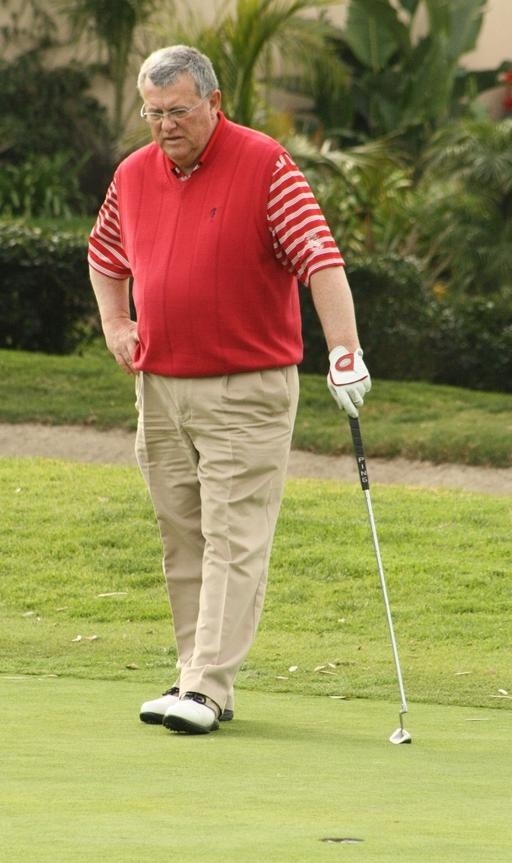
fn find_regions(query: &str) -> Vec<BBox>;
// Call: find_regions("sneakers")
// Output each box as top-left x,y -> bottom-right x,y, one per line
163,691 -> 221,733
138,687 -> 233,724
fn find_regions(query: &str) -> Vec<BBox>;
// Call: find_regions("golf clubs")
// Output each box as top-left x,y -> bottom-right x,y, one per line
347,414 -> 411,744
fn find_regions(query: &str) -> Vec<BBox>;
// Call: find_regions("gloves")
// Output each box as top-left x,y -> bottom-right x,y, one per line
326,345 -> 372,419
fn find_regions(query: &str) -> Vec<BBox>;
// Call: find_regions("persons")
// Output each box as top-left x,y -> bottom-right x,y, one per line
85,43 -> 375,734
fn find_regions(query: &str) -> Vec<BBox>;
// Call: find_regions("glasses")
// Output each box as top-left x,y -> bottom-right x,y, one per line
139,96 -> 205,122
